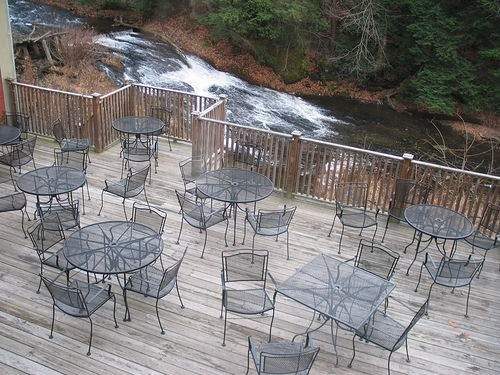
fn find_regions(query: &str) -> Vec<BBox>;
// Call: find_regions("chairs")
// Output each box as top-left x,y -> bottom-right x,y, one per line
0,107 -> 500,375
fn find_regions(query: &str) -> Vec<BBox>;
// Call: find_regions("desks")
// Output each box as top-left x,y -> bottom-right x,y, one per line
113,116 -> 164,173
274,253 -> 396,369
0,125 -> 21,174
196,169 -> 275,246
15,166 -> 86,238
405,205 -> 474,275
63,221 -> 164,322
